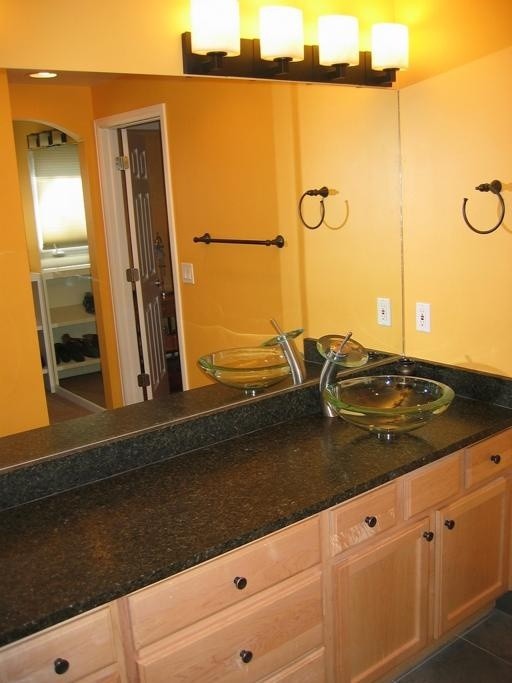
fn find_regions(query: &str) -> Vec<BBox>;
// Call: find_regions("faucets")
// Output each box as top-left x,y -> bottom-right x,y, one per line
270,318 -> 308,386
317,331 -> 352,419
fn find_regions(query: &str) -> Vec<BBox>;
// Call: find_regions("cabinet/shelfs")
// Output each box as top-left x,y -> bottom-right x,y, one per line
325,427 -> 511,683
31,262 -> 100,397
127,515 -> 327,683
1,591 -> 133,680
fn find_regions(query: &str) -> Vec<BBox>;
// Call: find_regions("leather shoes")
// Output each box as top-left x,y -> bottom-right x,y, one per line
55,333 -> 100,363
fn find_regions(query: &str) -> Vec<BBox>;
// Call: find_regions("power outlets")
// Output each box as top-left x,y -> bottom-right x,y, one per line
376,298 -> 391,328
414,302 -> 431,333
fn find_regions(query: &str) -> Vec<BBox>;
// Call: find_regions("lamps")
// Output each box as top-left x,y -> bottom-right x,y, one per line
181,0 -> 412,87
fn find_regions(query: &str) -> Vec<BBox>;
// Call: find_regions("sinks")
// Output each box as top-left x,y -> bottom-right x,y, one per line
198,346 -> 293,388
321,375 -> 456,435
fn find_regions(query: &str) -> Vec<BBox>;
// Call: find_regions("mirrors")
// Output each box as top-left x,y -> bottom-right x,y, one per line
0,65 -> 406,475
